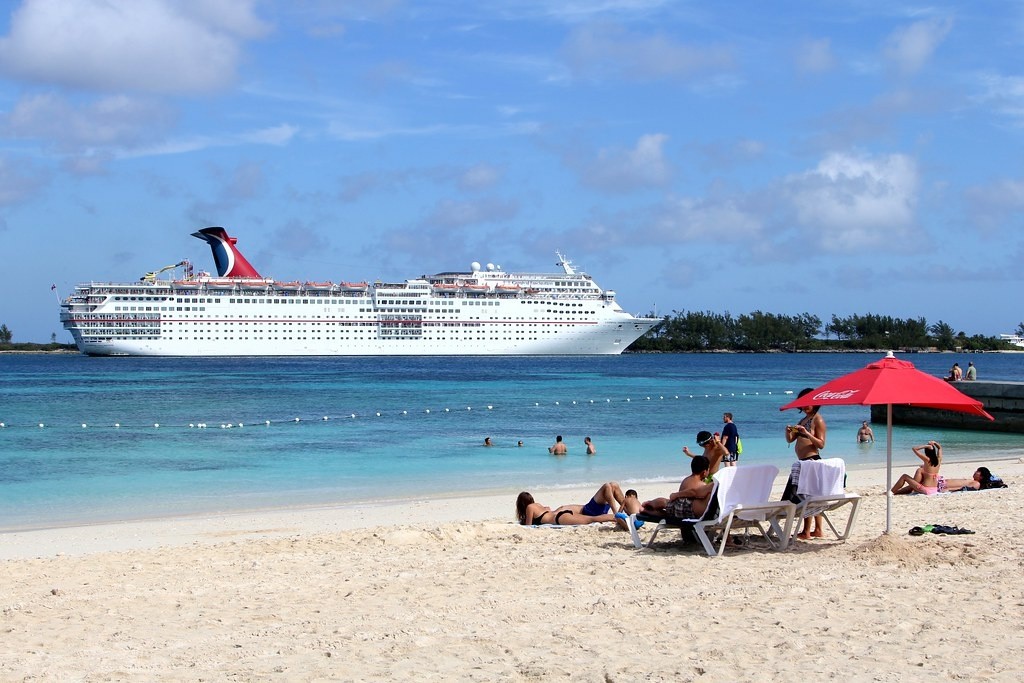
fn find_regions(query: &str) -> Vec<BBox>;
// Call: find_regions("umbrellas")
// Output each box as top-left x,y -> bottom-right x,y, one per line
779,351 -> 995,534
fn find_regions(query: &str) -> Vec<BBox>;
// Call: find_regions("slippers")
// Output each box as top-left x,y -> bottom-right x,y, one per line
909,527 -> 923,535
940,526 -> 951,532
932,525 -> 942,533
948,527 -> 959,534
923,525 -> 934,532
958,528 -> 975,534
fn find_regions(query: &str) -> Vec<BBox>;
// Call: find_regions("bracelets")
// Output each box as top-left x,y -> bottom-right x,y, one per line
715,440 -> 721,444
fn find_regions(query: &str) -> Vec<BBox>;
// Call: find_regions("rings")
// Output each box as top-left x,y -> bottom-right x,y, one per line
788,428 -> 790,430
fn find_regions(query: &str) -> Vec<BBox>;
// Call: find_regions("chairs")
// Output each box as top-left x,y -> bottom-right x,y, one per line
625,458 -> 862,556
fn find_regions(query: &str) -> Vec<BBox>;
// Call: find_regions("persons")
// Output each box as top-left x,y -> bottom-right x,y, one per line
883,440 -> 942,495
963,361 -> 976,381
785,388 -> 825,540
483,438 -> 494,446
547,436 -> 567,454
942,363 -> 965,381
584,437 -> 596,454
895,467 -> 991,494
517,412 -> 742,530
518,440 -> 523,446
857,420 -> 874,444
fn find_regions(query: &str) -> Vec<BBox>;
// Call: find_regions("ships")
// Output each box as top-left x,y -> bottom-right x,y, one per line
52,249 -> 667,356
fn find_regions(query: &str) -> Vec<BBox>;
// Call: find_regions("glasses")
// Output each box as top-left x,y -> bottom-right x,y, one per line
695,441 -> 705,446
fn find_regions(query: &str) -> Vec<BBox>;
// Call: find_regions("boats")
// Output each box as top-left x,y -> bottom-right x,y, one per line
168,278 -> 371,293
432,282 -> 522,295
